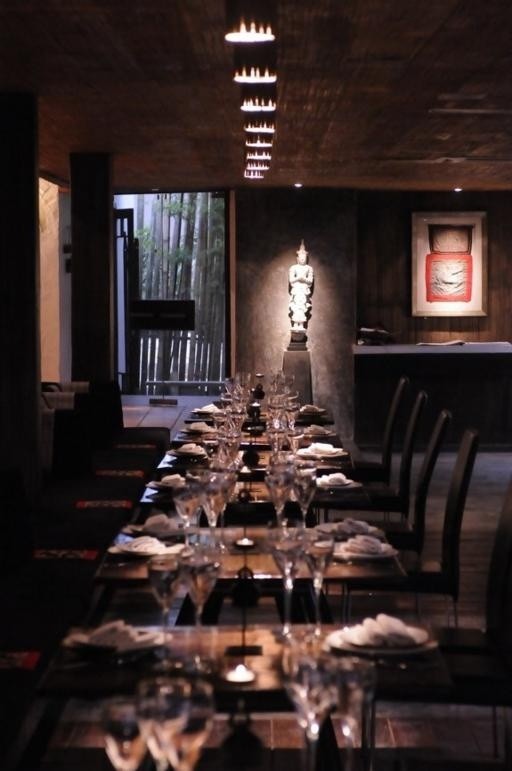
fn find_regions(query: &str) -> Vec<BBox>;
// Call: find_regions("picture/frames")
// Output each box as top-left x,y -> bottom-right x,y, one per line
410,211 -> 489,319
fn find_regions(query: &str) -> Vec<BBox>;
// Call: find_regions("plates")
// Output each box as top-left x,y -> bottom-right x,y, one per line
106,540 -> 186,561
312,520 -> 380,539
323,538 -> 401,564
118,518 -> 198,539
321,625 -> 444,659
57,625 -> 177,656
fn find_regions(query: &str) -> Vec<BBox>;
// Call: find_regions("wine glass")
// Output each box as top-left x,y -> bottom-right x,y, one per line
147,553 -> 196,649
170,481 -> 204,557
263,466 -> 293,541
291,465 -> 317,541
200,469 -> 227,555
139,369 -> 366,493
82,650 -> 383,771
175,543 -> 225,654
261,535 -> 307,642
299,531 -> 339,647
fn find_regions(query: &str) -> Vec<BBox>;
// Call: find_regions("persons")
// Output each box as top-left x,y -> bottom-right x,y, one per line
287,238 -> 315,335
